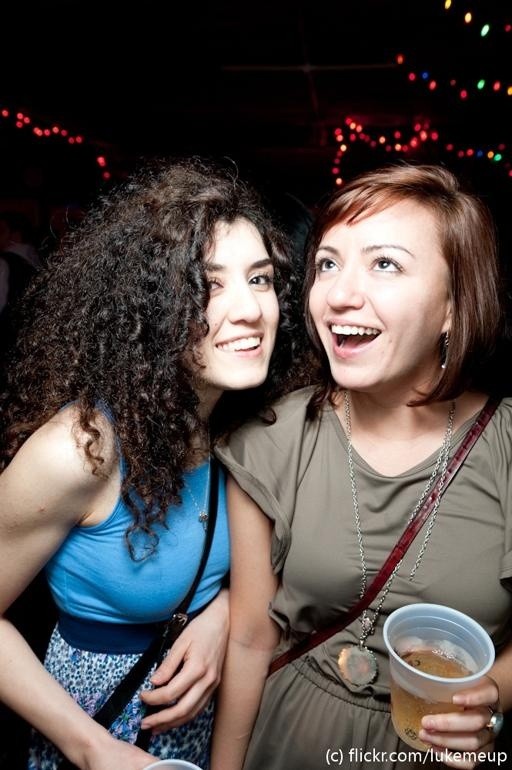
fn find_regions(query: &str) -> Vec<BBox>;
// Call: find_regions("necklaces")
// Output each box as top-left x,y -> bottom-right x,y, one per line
176,430 -> 212,532
334,390 -> 457,686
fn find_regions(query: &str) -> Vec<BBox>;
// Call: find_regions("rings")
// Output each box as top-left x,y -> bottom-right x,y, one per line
486,707 -> 505,737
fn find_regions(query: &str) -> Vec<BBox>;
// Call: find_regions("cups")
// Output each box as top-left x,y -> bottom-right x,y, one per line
382,603 -> 496,753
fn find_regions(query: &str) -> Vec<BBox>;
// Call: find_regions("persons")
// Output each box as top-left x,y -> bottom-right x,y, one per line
0,211 -> 47,313
1,155 -> 324,769
201,157 -> 512,770
